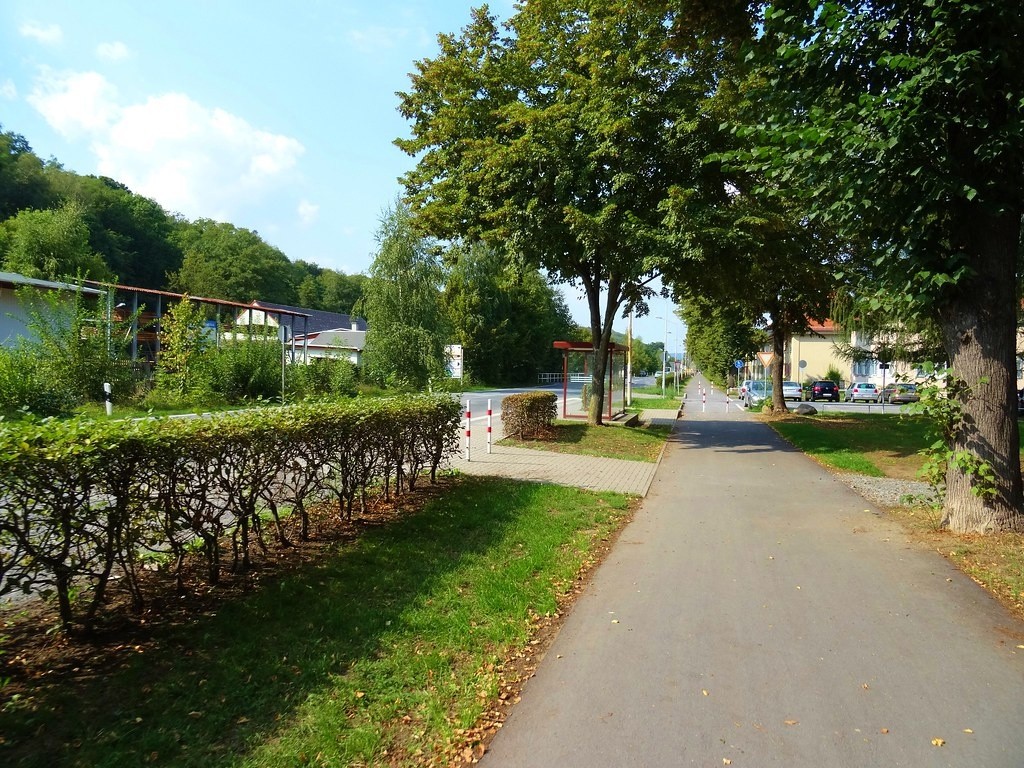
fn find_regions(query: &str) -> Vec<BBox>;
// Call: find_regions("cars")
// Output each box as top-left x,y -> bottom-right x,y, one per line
783,381 -> 802,402
843,383 -> 878,403
744,381 -> 773,408
1018,388 -> 1024,411
877,383 -> 920,403
805,380 -> 840,403
637,371 -> 646,377
738,380 -> 764,400
655,370 -> 663,379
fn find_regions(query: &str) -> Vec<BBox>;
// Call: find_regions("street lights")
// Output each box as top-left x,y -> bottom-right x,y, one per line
653,296 -> 667,398
108,303 -> 125,358
656,316 -> 690,393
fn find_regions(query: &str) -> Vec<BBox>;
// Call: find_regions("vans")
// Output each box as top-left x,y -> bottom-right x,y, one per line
665,367 -> 672,374
